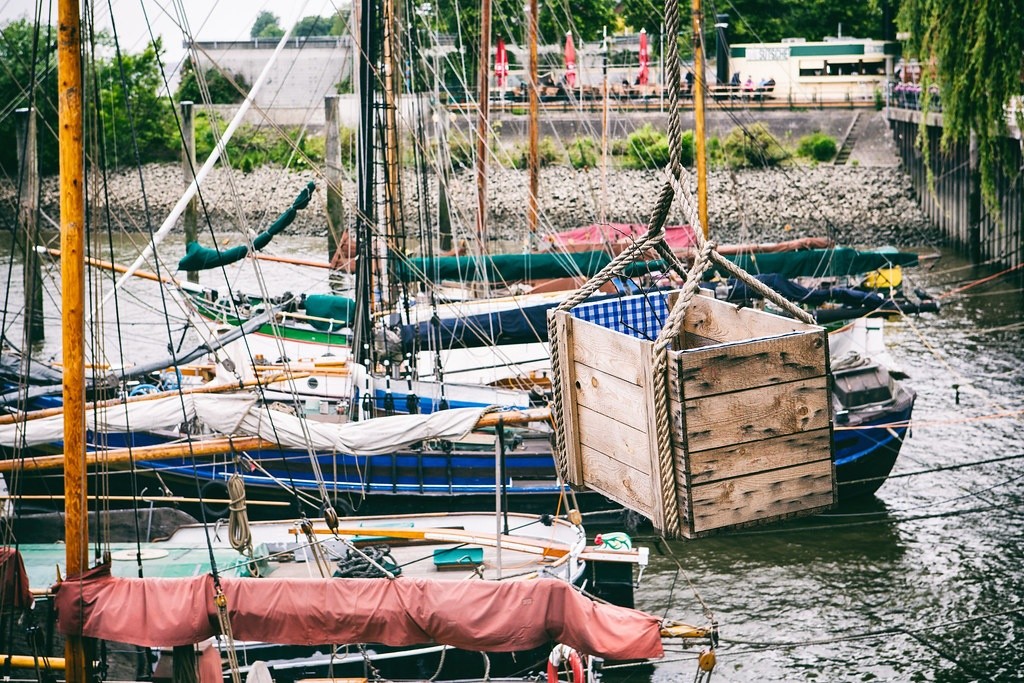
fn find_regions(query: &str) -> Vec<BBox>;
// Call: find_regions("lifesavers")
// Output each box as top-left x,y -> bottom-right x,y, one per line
547,643 -> 585,683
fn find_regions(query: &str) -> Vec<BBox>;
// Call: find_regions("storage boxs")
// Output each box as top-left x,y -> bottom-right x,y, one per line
547,289 -> 839,542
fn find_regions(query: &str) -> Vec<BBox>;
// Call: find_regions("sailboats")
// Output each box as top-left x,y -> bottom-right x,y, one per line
0,0 -> 937,682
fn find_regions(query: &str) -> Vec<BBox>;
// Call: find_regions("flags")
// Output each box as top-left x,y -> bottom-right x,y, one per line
564,35 -> 577,94
636,33 -> 649,85
493,38 -> 508,87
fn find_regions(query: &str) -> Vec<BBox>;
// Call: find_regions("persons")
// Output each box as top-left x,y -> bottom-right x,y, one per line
685,70 -> 695,89
732,71 -> 775,102
